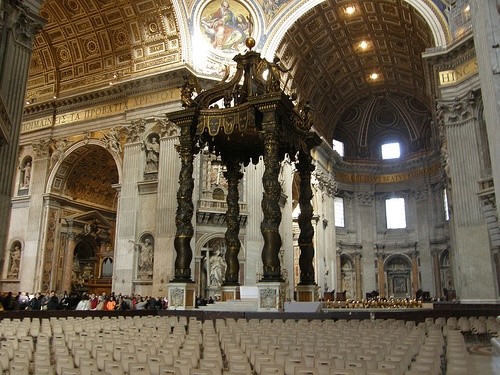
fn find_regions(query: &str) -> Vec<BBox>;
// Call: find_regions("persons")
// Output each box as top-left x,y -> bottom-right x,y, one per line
0,289 -> 222,312
202,0 -> 249,52
319,286 -> 456,310
7,245 -> 21,274
20,161 -> 31,187
143,136 -> 161,174
128,237 -> 154,279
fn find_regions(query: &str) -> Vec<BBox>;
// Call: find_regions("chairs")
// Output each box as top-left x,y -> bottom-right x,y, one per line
0,315 -> 499,375
